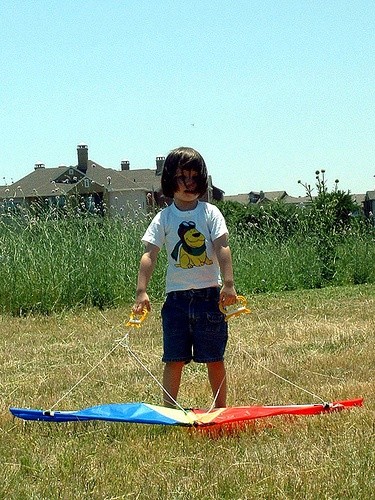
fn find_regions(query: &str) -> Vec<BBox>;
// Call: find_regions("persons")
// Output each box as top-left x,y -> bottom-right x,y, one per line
133,146 -> 238,409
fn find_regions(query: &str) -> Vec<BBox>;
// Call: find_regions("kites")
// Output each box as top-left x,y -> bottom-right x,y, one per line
7,398 -> 365,428
